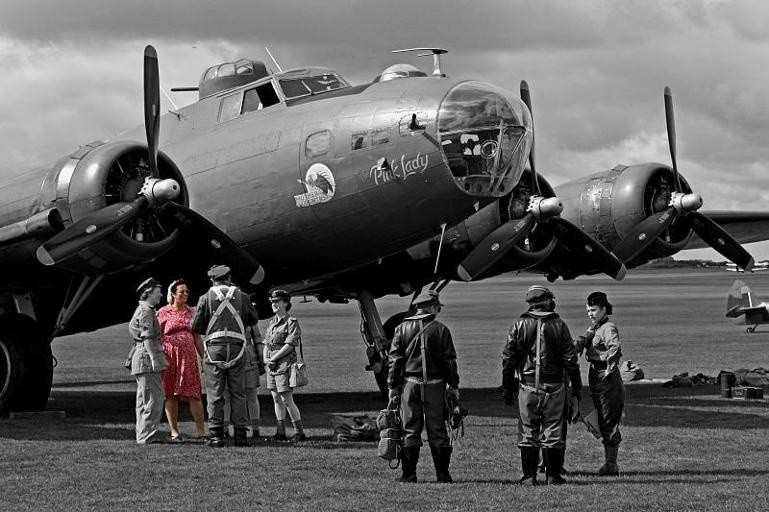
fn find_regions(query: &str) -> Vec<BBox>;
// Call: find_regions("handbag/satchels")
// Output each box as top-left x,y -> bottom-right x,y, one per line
375,399 -> 403,470
288,362 -> 310,387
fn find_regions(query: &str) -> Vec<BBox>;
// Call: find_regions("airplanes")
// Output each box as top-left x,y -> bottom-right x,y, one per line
0,43 -> 769,413
722,259 -> 768,274
718,277 -> 769,338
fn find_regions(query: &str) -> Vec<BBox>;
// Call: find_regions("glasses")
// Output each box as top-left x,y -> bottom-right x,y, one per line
176,289 -> 189,294
436,307 -> 441,313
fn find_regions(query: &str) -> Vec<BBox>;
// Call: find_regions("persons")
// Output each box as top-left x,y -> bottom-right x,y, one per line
536,326 -> 595,471
220,324 -> 264,440
156,276 -> 203,438
498,284 -> 581,481
262,289 -> 305,442
193,265 -> 258,445
129,276 -> 168,442
389,288 -> 460,482
584,292 -> 627,475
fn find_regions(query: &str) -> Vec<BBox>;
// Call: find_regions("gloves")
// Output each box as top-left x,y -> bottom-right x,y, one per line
568,396 -> 582,424
502,386 -> 516,408
597,364 -> 616,381
387,386 -> 402,404
447,385 -> 461,401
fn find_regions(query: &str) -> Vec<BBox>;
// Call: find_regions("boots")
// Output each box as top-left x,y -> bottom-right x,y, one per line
429,445 -> 454,484
398,445 -> 422,483
516,445 -> 541,486
207,426 -> 225,449
233,426 -> 250,447
598,445 -> 619,475
269,417 -> 287,441
541,447 -> 569,485
288,417 -> 306,444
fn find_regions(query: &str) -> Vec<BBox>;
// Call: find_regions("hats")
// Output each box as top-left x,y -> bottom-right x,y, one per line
136,277 -> 162,297
525,284 -> 556,302
587,292 -> 609,307
269,290 -> 292,301
207,264 -> 231,281
412,290 -> 444,307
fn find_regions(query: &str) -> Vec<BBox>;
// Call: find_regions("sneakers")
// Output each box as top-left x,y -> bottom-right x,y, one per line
223,430 -> 231,439
252,429 -> 261,440
